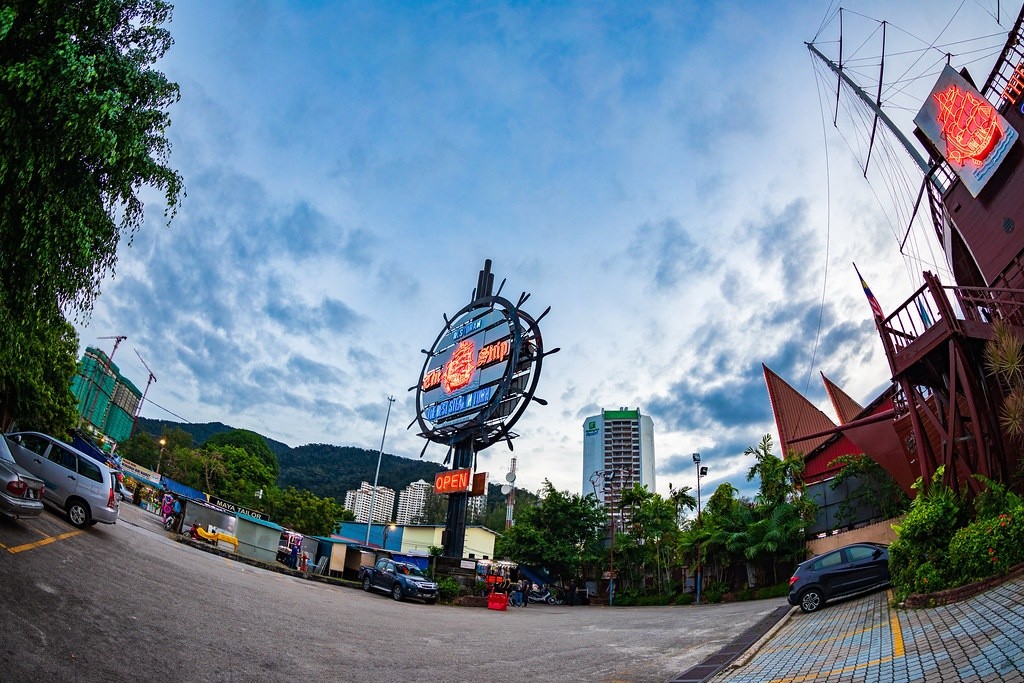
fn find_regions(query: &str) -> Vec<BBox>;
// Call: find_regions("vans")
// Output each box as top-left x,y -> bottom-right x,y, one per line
4,431 -> 120,529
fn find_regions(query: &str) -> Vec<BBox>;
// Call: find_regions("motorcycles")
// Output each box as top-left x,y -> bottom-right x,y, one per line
163,509 -> 178,531
526,585 -> 558,605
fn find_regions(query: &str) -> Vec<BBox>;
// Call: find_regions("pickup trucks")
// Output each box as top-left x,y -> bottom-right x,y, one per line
358,558 -> 440,607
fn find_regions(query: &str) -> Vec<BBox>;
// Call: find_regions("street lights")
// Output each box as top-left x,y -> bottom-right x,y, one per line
366,395 -> 396,549
693,453 -> 708,607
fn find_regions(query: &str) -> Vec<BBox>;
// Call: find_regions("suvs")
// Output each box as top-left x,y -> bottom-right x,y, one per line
787,542 -> 894,614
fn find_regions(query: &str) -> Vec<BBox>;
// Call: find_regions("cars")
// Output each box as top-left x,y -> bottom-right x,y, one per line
0,427 -> 45,523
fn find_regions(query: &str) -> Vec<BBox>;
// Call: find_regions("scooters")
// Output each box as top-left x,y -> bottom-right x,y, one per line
181,519 -> 215,547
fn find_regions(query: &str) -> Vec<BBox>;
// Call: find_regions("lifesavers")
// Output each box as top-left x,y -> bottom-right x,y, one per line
164,517 -> 174,530
163,505 -> 171,513
174,502 -> 181,513
165,495 -> 173,504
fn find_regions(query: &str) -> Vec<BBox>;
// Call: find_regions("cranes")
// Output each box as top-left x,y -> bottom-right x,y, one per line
131,346 -> 158,435
88,334 -> 127,427
